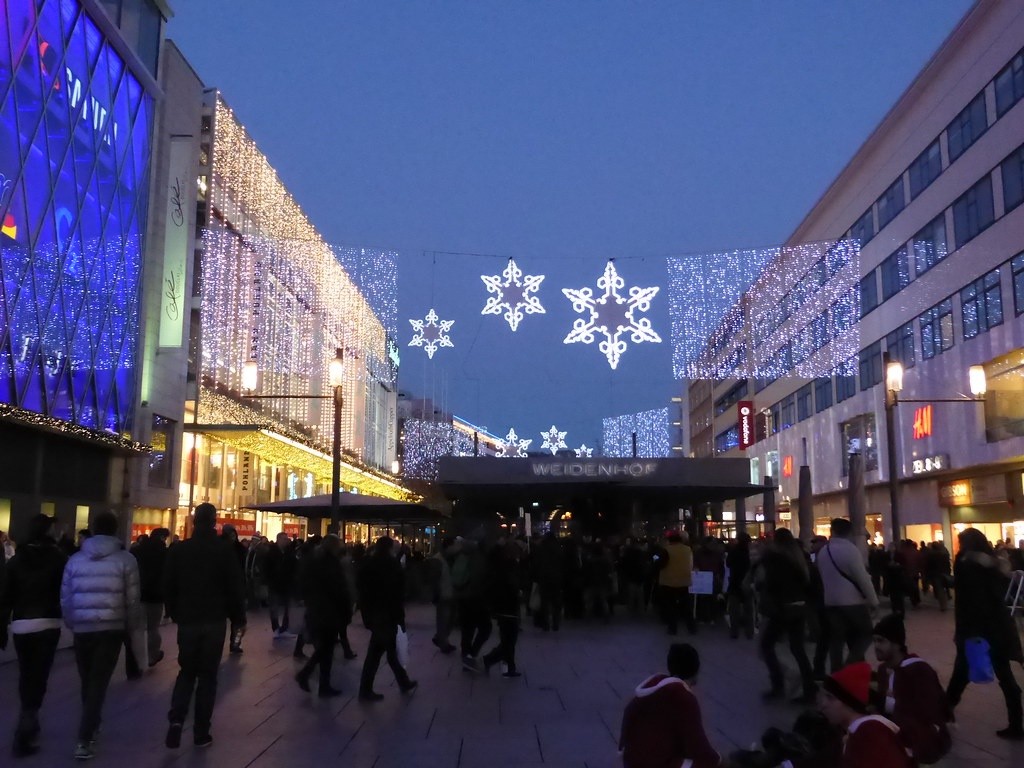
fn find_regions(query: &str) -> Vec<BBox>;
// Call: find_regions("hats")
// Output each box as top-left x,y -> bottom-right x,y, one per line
819,662 -> 872,712
667,644 -> 700,675
664,530 -> 679,538
30,514 -> 56,538
873,614 -> 905,645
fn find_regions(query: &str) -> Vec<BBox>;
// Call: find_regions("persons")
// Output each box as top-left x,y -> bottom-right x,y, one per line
239,531 -> 425,659
617,640 -> 723,768
649,528 -> 699,635
945,528 -> 1024,741
59,512 -> 140,759
157,501 -> 235,748
218,520 -> 249,655
426,526 -> 759,679
297,535 -> 353,699
353,537 -> 420,702
0,513 -> 185,760
755,518 -> 1024,768
264,529 -> 299,639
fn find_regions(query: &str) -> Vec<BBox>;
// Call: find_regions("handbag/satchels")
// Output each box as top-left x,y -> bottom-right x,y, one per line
965,634 -> 995,684
731,710 -> 833,768
528,582 -> 541,612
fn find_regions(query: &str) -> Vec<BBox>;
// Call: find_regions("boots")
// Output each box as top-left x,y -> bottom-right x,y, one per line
763,673 -> 785,698
791,682 -> 817,706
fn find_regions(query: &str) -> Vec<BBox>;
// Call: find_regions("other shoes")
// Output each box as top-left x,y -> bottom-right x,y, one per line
165,721 -> 181,748
11,719 -> 41,756
128,671 -> 142,681
74,731 -> 97,759
294,636 -> 521,703
149,650 -> 163,666
194,734 -> 212,747
996,724 -> 1024,740
229,643 -> 243,653
813,670 -> 826,680
273,629 -> 297,639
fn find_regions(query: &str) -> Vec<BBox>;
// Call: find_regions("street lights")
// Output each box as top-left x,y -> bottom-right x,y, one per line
242,348 -> 344,535
884,351 -> 987,548
391,461 -> 435,486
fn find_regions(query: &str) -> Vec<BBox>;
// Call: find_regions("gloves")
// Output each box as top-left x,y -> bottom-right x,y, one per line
0,640 -> 7,651
